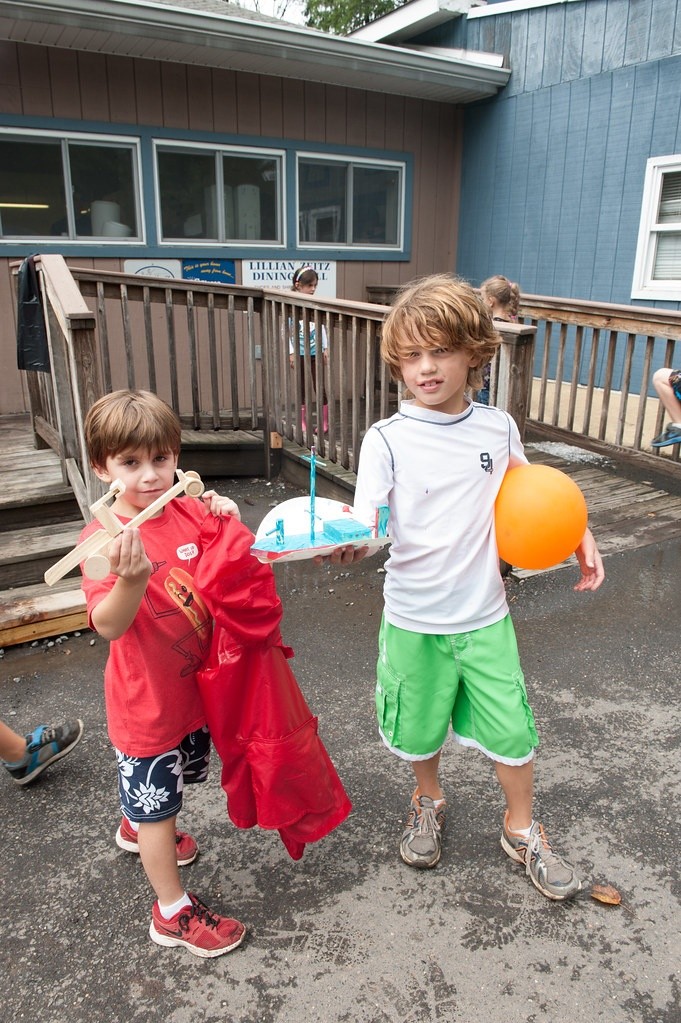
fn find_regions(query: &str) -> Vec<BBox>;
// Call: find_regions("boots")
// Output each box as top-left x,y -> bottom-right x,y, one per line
301,405 -> 306,431
315,404 -> 328,433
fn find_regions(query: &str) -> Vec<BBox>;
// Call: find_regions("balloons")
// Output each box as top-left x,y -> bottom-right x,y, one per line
494,463 -> 588,570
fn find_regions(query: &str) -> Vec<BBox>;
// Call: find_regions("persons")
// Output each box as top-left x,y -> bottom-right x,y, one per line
281,266 -> 328,433
0,718 -> 83,785
315,274 -> 604,900
651,368 -> 681,447
77,389 -> 245,958
474,275 -> 520,405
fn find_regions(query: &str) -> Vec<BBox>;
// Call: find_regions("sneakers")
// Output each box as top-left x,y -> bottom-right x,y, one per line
3,718 -> 84,784
650,422 -> 681,447
400,785 -> 446,868
500,810 -> 581,900
149,893 -> 246,958
116,817 -> 198,866
